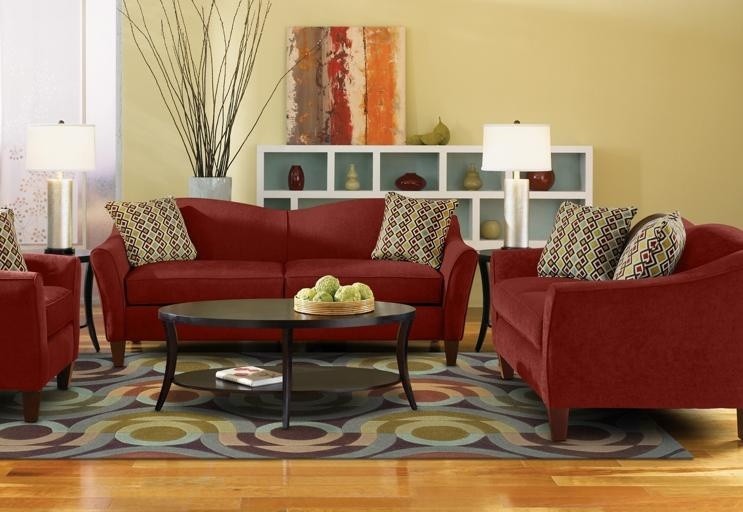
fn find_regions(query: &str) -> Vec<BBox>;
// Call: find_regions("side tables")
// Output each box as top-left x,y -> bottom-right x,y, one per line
475,249 -> 498,352
74,248 -> 100,353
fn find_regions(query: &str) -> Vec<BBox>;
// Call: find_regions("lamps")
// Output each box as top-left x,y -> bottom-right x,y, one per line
481,123 -> 552,249
27,123 -> 95,255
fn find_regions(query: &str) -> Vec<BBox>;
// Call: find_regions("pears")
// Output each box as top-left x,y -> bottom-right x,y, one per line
406,116 -> 450,145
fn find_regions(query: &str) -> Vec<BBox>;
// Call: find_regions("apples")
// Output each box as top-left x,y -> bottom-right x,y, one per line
296,276 -> 372,302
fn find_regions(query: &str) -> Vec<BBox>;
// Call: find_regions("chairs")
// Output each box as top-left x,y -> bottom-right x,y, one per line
0,253 -> 81,423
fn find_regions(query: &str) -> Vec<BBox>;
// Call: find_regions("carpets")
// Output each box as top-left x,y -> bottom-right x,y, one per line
0,351 -> 694,461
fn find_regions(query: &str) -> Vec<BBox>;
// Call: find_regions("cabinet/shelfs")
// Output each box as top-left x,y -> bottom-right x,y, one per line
256,144 -> 593,252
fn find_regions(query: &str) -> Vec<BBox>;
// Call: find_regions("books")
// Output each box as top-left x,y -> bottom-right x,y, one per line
215,365 -> 283,387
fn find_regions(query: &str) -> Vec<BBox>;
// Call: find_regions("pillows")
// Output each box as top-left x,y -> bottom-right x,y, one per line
0,207 -> 29,274
612,210 -> 686,279
371,192 -> 458,270
538,200 -> 637,283
104,194 -> 197,267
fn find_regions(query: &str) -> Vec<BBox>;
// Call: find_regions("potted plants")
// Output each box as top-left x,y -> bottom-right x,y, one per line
119,0 -> 326,201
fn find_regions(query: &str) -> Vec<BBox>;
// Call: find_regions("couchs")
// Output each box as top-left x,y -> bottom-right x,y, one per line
90,197 -> 480,369
489,214 -> 743,443
